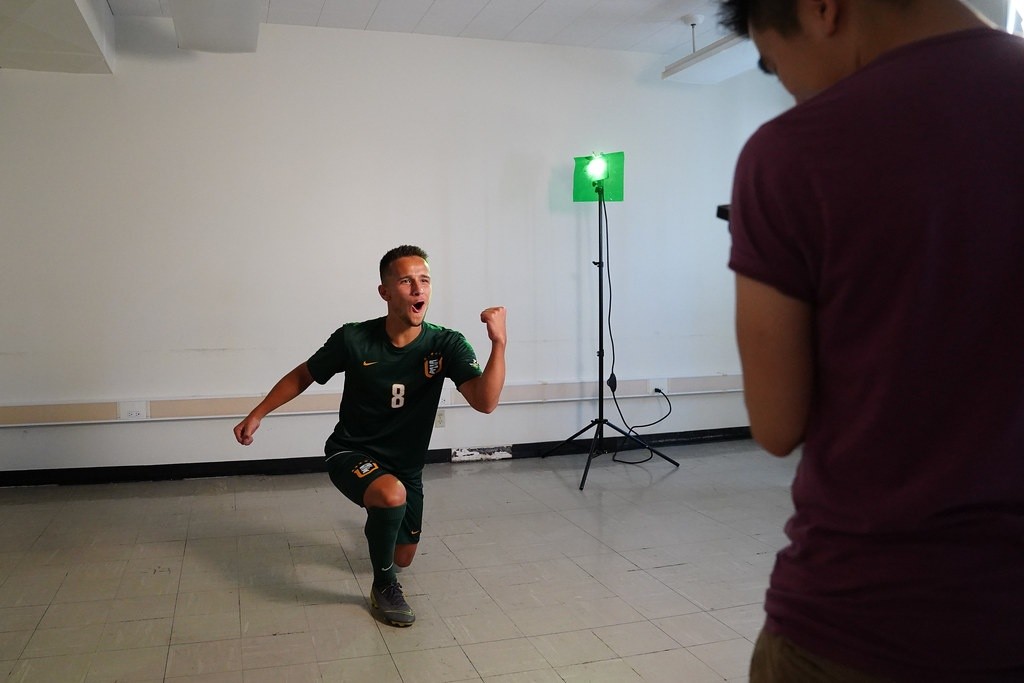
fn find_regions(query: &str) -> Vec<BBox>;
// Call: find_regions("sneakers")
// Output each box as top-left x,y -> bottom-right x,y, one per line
368,578 -> 415,627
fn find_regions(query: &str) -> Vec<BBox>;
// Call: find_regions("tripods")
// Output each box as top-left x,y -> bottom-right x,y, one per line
542,182 -> 680,489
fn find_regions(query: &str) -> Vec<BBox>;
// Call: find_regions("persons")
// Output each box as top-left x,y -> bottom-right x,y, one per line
231,245 -> 509,627
720,1 -> 1022,683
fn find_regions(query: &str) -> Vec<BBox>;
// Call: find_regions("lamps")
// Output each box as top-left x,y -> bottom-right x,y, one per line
660,12 -> 746,80
